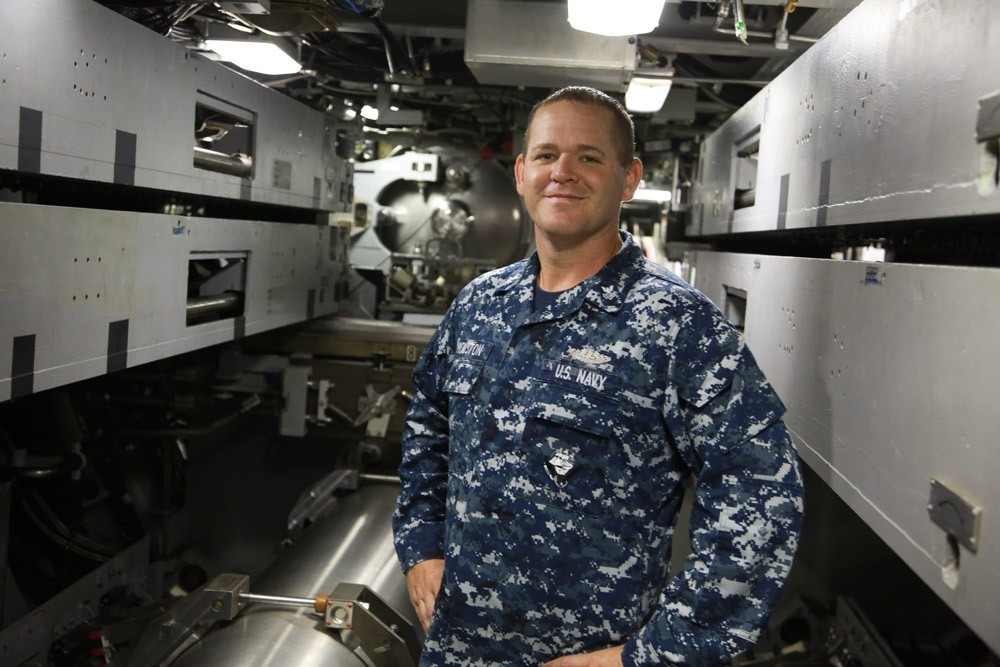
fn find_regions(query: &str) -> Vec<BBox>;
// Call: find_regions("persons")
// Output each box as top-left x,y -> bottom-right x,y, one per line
394,86 -> 802,667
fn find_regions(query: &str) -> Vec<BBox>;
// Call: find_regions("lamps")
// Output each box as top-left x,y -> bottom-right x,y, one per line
195,19 -> 306,75
622,56 -> 679,115
